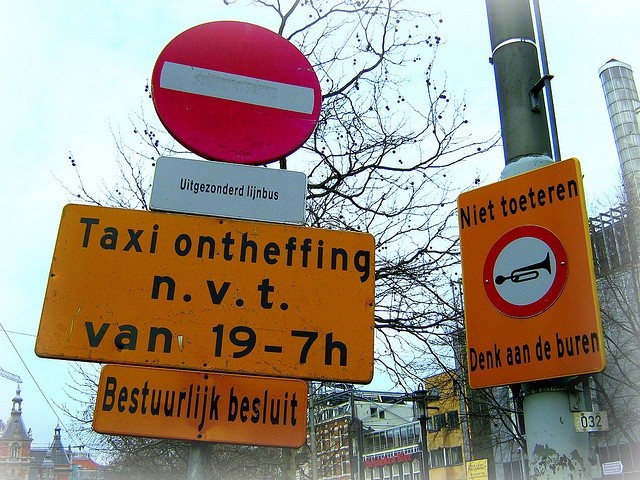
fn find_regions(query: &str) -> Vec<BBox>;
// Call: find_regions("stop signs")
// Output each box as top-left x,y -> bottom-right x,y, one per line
150,20 -> 322,165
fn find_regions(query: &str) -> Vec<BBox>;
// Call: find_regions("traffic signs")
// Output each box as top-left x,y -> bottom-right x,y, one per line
602,461 -> 623,476
90,363 -> 309,449
456,158 -> 606,391
148,156 -> 308,228
34,203 -> 376,386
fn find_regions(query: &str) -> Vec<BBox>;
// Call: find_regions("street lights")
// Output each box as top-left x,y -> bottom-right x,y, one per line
411,382 -> 432,480
